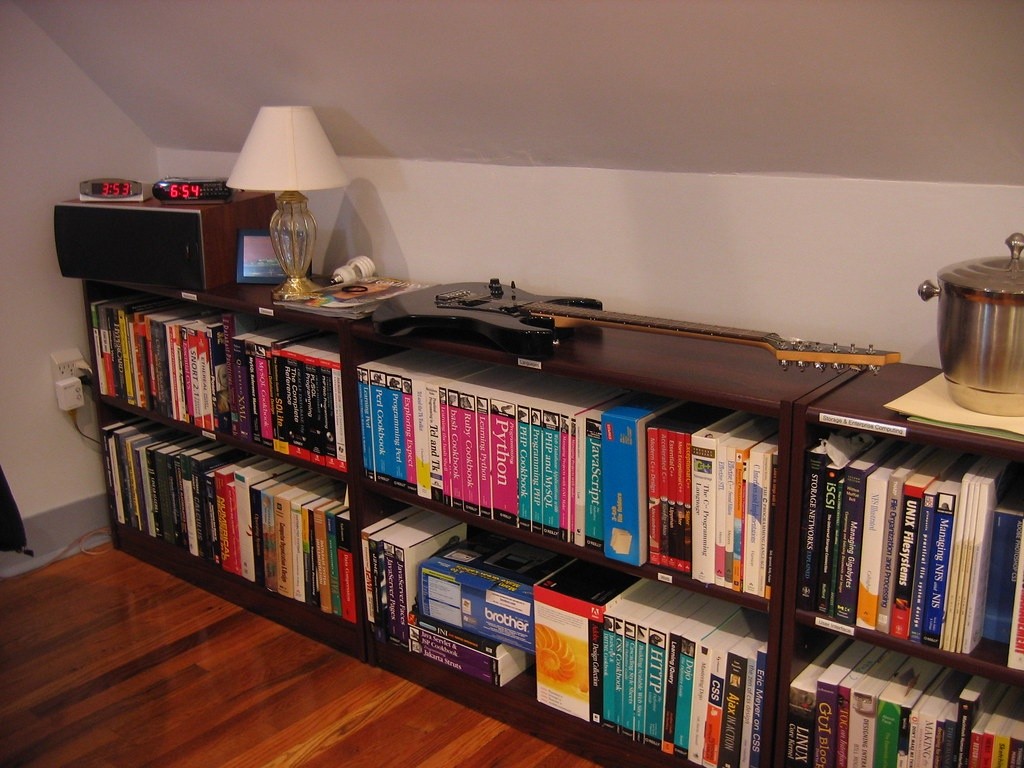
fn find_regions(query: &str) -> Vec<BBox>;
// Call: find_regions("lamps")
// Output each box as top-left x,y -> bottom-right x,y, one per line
226,106 -> 352,301
330,255 -> 377,286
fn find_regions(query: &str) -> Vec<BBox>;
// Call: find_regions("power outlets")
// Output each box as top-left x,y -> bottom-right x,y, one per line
51,348 -> 85,382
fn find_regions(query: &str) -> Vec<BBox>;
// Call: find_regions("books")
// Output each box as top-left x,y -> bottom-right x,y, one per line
283,275 -> 440,319
92,301 -> 1024,768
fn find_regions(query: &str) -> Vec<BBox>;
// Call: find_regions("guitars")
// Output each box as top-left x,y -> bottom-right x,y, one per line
370,280 -> 903,376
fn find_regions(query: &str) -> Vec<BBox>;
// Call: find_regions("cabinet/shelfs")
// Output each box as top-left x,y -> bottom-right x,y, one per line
771,361 -> 1024,768
351,290 -> 873,768
81,278 -> 444,664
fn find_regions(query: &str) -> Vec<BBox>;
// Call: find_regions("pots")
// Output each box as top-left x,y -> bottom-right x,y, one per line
917,232 -> 1024,417
